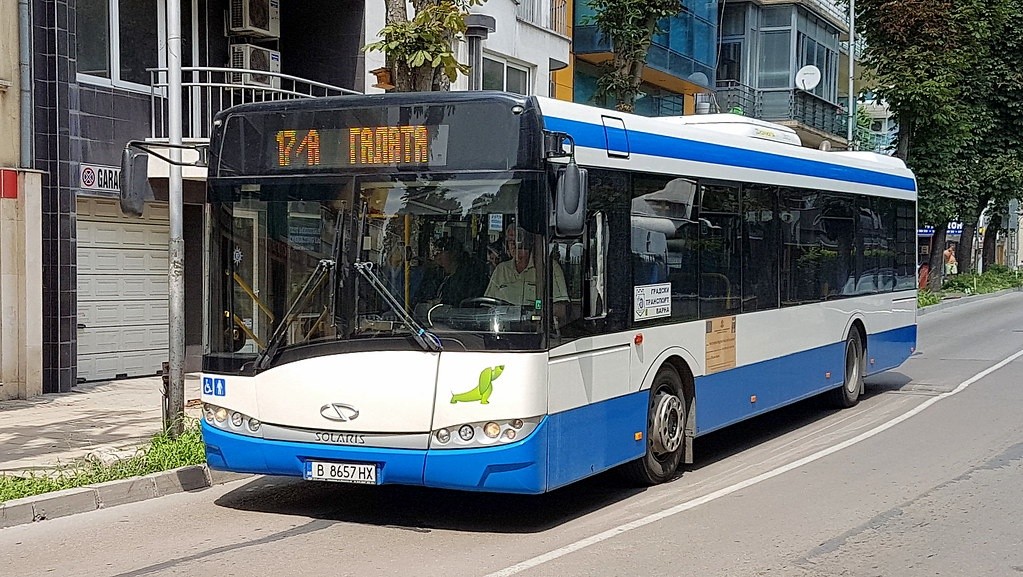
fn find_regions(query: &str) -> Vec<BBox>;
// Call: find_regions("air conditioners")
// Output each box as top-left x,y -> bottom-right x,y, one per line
228,44 -> 281,93
223,0 -> 280,39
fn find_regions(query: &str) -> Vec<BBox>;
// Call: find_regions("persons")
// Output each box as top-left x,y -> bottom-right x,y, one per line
380,223 -> 570,319
944,243 -> 958,277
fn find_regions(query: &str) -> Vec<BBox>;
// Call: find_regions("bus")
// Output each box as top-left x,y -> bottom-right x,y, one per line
118,90 -> 921,495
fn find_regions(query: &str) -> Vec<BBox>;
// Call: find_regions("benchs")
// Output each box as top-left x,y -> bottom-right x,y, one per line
667,271 -> 732,313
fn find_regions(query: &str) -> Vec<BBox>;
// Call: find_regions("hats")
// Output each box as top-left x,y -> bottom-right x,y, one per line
432,235 -> 464,255
